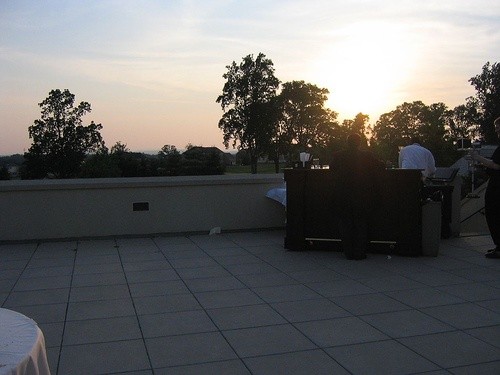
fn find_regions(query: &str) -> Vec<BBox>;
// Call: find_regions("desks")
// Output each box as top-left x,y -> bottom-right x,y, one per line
0,308 -> 51,375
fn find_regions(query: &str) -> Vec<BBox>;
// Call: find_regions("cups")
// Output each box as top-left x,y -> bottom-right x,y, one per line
309,158 -> 320,170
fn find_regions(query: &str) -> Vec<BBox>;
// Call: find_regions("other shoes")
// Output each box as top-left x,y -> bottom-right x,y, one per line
487,247 -> 500,253
485,250 -> 500,259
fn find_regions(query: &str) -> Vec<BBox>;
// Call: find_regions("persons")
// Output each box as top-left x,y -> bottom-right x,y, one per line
329,133 -> 387,169
398,137 -> 436,169
471,116 -> 500,260
